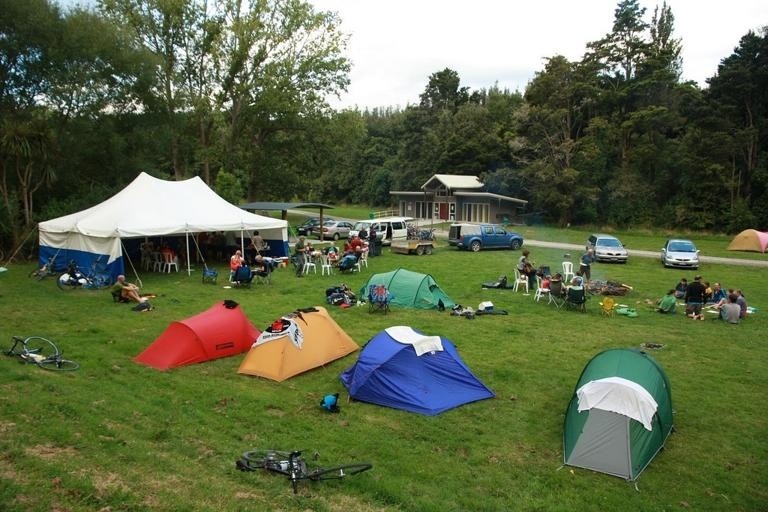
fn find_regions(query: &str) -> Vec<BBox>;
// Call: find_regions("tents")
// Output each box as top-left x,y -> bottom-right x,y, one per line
725,228 -> 768,254
359,266 -> 454,310
133,299 -> 262,371
338,325 -> 496,416
561,347 -> 673,481
237,306 -> 359,383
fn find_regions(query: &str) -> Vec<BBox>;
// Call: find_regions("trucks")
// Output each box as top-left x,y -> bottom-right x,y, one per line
448,222 -> 524,252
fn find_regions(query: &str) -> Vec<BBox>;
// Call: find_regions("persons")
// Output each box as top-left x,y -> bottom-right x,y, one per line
503,215 -> 509,228
111,275 -> 145,304
657,274 -> 747,323
295,224 -> 376,278
386,222 -> 392,239
141,231 -> 270,289
517,248 -> 595,305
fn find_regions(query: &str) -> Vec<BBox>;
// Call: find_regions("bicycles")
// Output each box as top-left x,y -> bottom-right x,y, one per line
236,447 -> 373,494
29,257 -> 53,279
59,263 -> 109,289
2,334 -> 81,372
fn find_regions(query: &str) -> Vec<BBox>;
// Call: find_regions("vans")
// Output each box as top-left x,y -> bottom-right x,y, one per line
349,216 -> 414,247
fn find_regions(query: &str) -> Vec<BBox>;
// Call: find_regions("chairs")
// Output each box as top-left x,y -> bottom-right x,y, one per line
254,259 -> 272,287
202,262 -> 218,284
228,268 -> 237,283
564,285 -> 587,314
328,247 -> 340,267
512,268 -> 529,294
361,251 -> 368,268
562,262 -> 574,282
548,279 -> 566,309
350,258 -> 361,273
303,254 -> 316,275
533,275 -> 553,305
140,244 -> 179,273
319,254 -> 332,276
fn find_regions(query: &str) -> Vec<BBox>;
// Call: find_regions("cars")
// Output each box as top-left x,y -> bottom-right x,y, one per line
298,217 -> 334,233
661,240 -> 701,270
586,233 -> 628,266
314,221 -> 355,240
